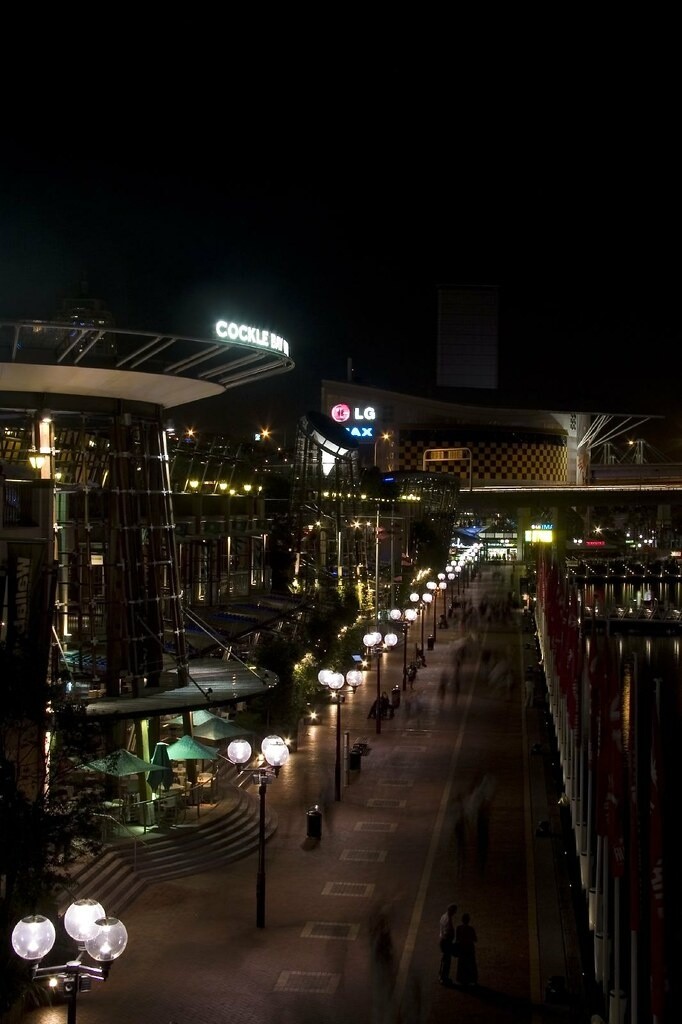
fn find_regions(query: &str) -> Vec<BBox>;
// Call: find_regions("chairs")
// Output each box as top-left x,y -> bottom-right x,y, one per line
112,760 -> 213,822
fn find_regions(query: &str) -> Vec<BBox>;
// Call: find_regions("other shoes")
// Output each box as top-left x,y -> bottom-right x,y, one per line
439,976 -> 453,985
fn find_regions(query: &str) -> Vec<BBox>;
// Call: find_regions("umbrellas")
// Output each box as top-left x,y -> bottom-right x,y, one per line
87,705 -> 255,779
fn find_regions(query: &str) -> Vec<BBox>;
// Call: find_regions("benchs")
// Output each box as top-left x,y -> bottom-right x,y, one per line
353,735 -> 370,756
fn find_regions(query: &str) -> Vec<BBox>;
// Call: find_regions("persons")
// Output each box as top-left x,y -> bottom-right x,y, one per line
436,903 -> 457,986
475,798 -> 490,879
454,807 -> 467,878
439,567 -> 516,704
408,661 -> 417,689
455,914 -> 479,985
372,918 -> 399,1000
368,691 -> 389,719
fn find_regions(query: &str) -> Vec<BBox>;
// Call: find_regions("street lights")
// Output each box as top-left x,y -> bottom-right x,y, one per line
438,573 -> 455,622
361,630 -> 399,735
425,581 -> 447,643
390,607 -> 417,690
445,541 -> 485,608
12,898 -> 129,1024
318,666 -> 365,802
227,733 -> 291,927
410,593 -> 433,656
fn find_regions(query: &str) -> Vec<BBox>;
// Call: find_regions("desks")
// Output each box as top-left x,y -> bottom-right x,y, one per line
172,767 -> 187,777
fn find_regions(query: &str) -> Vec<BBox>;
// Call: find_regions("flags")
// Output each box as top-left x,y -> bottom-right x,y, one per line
545,559 -> 666,1015
